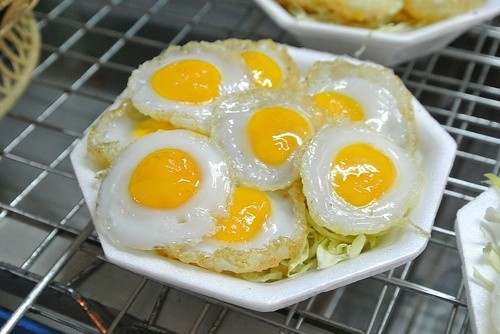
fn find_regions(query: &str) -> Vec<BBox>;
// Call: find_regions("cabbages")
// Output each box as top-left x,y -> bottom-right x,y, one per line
249,207 -> 389,287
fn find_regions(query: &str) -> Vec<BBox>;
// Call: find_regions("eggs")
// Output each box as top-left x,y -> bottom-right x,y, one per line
89,37 -> 419,270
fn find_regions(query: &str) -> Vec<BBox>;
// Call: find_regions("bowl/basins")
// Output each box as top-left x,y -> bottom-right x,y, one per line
258,0 -> 500,65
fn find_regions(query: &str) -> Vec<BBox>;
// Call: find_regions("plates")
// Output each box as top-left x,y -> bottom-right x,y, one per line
70,38 -> 460,313
